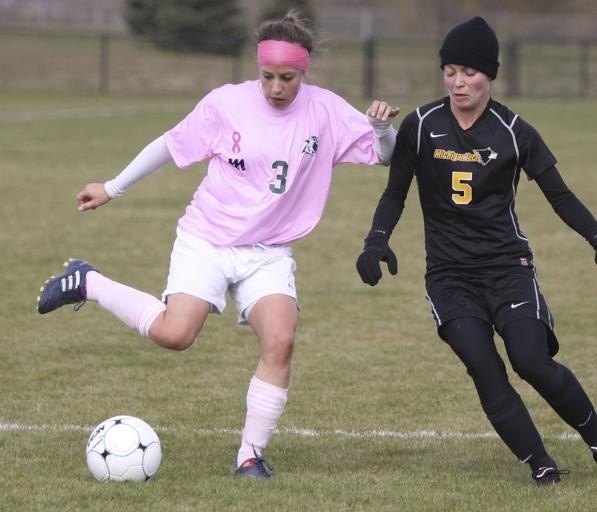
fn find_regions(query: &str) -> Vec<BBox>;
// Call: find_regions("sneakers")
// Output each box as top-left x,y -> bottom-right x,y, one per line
233,452 -> 274,483
34,255 -> 100,313
531,457 -> 561,485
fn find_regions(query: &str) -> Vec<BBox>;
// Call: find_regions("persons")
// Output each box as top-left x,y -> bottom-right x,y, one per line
36,7 -> 400,480
357,14 -> 597,485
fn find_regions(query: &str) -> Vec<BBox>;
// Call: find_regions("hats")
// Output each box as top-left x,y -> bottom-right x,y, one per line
438,12 -> 501,83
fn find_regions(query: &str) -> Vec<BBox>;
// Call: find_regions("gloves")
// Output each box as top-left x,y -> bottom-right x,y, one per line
356,234 -> 398,286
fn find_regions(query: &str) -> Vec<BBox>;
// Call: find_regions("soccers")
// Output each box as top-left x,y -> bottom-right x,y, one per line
86,415 -> 161,484
